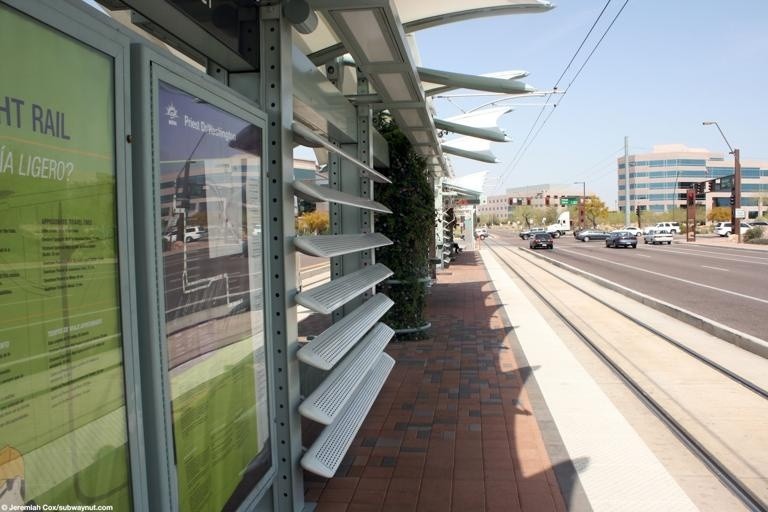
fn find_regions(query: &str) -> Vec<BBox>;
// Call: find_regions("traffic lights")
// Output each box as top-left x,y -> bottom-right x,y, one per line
689,192 -> 694,206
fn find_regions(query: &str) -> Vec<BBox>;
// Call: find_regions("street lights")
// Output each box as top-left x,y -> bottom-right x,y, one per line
573,181 -> 586,228
702,119 -> 742,243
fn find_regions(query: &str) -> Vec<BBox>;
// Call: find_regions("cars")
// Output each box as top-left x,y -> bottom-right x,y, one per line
165,222 -> 261,244
689,219 -> 768,239
460,227 -> 488,239
519,220 -> 683,249
